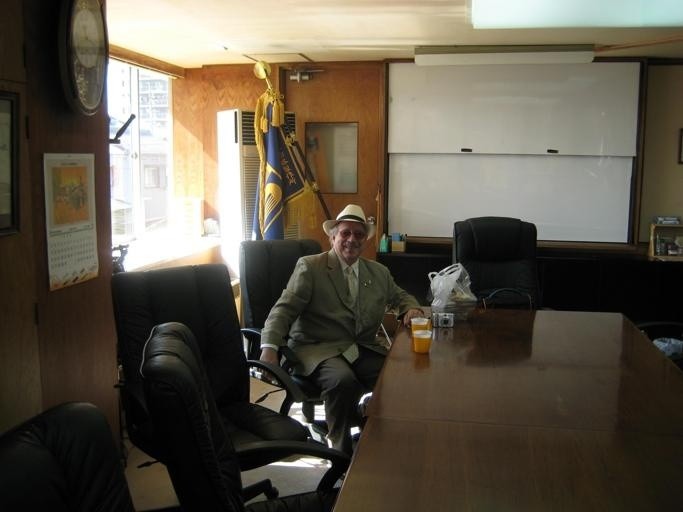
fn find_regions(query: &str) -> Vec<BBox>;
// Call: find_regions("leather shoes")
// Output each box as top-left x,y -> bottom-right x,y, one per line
356,404 -> 368,431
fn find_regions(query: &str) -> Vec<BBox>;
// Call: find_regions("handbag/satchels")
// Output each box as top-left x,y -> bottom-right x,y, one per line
482,287 -> 534,309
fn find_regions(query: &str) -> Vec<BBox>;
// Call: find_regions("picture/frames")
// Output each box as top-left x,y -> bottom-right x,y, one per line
679,129 -> 683,164
0,91 -> 21,238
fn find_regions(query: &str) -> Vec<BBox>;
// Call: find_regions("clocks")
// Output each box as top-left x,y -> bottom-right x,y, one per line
36,0 -> 109,116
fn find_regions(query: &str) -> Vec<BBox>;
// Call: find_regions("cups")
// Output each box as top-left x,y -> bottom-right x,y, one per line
410,317 -> 432,355
380,239 -> 388,253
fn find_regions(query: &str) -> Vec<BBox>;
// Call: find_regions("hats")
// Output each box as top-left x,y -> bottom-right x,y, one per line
323,204 -> 376,241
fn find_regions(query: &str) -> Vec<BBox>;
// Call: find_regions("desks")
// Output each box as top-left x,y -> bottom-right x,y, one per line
375,239 -> 683,324
332,310 -> 683,511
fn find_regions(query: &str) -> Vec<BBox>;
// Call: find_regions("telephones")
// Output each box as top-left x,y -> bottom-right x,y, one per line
112,244 -> 128,274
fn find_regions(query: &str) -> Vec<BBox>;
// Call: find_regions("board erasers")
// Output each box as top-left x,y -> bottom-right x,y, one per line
392,232 -> 400,241
461,148 -> 473,152
546,149 -> 559,152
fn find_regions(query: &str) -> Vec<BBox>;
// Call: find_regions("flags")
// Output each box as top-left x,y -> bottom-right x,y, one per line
252,86 -> 320,240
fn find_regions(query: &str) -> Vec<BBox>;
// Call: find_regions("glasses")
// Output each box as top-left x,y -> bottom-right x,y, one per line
340,230 -> 365,239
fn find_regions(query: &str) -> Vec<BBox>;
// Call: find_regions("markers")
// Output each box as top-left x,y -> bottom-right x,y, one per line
381,233 -> 388,241
399,233 -> 407,241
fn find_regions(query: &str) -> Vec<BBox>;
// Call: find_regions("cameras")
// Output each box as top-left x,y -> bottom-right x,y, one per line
432,327 -> 453,342
432,312 -> 454,328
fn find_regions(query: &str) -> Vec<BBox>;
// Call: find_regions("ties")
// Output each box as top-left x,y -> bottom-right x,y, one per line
342,265 -> 360,364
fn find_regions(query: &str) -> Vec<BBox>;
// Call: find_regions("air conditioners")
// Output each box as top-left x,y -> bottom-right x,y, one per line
217,108 -> 305,278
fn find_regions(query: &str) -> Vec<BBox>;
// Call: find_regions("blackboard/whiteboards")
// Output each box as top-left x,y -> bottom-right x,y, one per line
386,61 -> 641,153
383,153 -> 643,249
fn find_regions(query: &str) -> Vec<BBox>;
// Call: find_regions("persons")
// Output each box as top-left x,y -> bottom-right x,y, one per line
259,221 -> 425,456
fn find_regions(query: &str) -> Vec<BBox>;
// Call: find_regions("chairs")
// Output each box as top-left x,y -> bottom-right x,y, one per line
0,401 -> 213,510
236,240 -> 325,421
636,319 -> 683,371
138,322 -> 351,512
452,217 -> 554,311
110,262 -> 309,504
